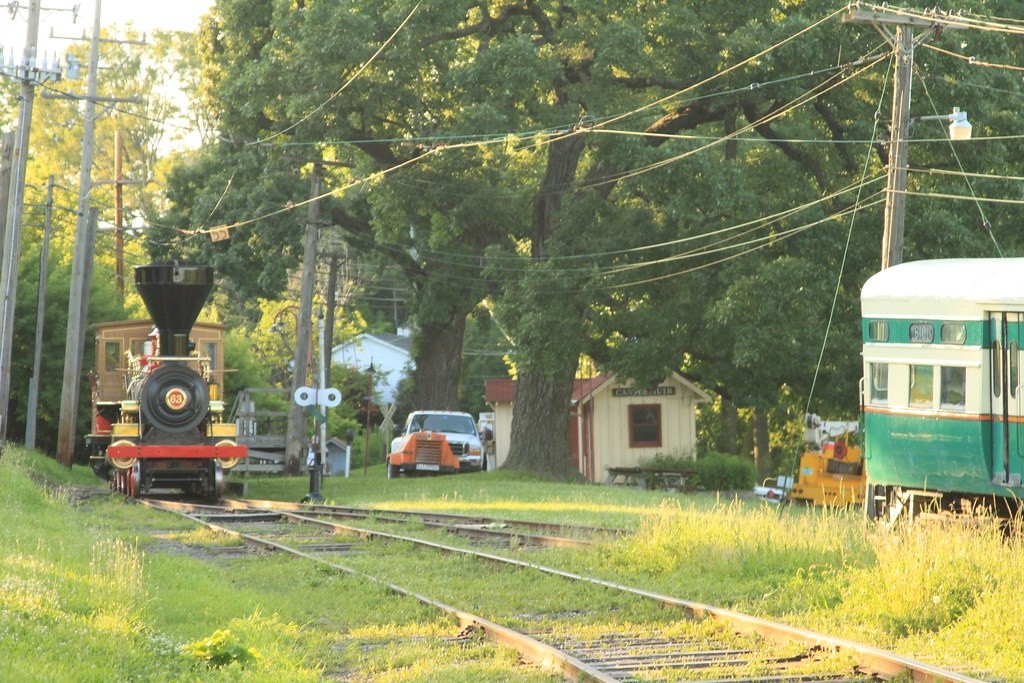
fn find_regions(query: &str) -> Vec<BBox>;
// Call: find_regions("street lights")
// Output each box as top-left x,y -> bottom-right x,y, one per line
878,109 -> 973,271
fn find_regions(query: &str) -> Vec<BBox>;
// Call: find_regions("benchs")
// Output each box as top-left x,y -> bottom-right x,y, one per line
603,467 -> 698,490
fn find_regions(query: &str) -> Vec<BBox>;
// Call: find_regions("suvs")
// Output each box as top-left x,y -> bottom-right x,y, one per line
387,409 -> 488,481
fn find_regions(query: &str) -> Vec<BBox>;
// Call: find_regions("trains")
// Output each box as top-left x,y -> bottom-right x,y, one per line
80,262 -> 247,505
